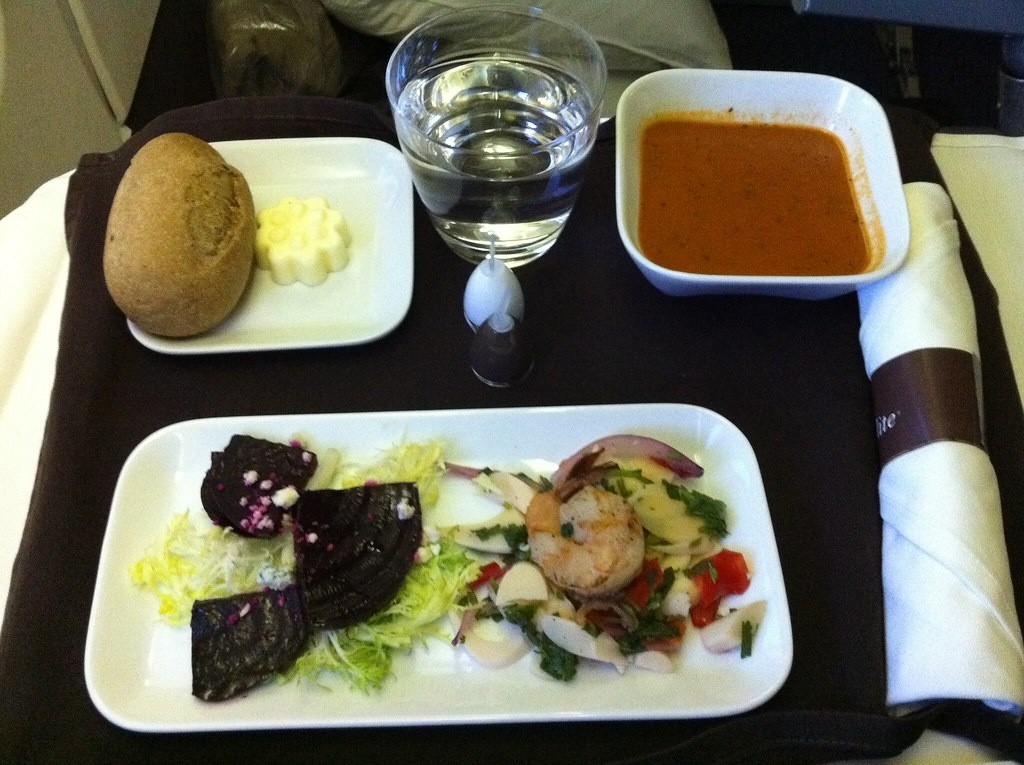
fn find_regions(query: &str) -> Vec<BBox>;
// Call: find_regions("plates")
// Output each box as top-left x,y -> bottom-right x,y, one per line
127,137 -> 415,355
83,403 -> 794,733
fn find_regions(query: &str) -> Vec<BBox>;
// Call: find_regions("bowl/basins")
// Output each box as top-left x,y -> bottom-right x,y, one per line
616,67 -> 911,299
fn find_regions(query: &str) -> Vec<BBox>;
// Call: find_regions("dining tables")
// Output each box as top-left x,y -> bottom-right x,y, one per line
1,99 -> 1024,763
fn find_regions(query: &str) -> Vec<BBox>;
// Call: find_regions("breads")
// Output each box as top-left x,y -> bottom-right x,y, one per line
104,133 -> 255,337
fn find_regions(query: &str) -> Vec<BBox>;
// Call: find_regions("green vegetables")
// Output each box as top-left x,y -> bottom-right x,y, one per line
129,435 -> 752,695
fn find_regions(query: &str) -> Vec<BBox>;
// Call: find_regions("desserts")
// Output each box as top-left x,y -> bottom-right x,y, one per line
255,196 -> 351,287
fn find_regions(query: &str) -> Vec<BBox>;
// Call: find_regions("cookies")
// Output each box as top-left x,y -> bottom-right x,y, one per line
291,481 -> 421,629
191,584 -> 308,703
199,434 -> 318,539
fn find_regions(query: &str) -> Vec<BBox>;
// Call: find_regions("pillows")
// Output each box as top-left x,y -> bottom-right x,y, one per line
325,0 -> 736,85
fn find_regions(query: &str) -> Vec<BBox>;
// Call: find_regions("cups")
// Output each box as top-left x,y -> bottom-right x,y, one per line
385,3 -> 606,269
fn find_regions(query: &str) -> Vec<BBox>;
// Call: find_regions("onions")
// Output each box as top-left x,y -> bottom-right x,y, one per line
549,435 -> 704,485
